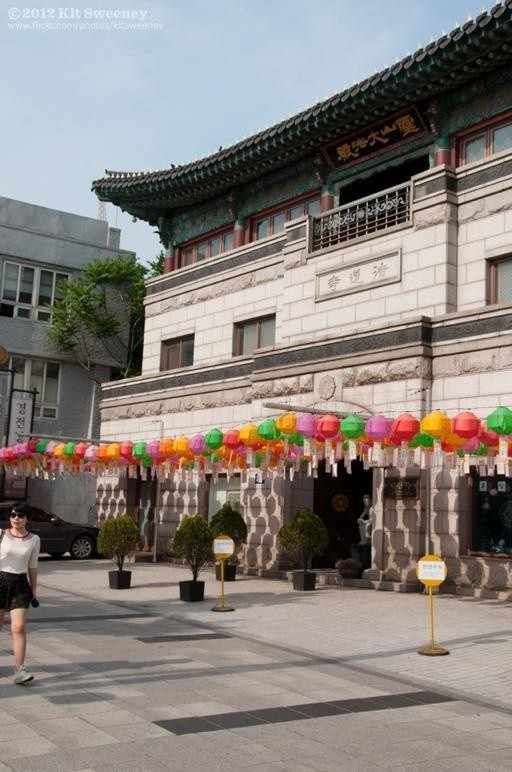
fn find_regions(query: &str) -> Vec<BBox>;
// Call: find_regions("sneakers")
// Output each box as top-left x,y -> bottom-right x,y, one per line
13,665 -> 35,684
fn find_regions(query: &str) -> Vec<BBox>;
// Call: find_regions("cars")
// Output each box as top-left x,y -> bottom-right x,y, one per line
0,499 -> 103,559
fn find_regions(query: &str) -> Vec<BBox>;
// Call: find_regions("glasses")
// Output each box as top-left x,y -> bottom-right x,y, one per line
9,512 -> 27,519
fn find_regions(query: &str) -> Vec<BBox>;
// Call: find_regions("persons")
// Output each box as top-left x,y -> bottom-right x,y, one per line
0,502 -> 41,684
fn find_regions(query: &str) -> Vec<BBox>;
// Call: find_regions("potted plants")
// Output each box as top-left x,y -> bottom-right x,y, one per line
168,512 -> 214,602
277,507 -> 331,592
96,513 -> 141,592
210,500 -> 251,581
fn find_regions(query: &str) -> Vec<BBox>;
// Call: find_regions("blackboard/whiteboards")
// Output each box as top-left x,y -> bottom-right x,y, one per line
467,464 -> 511,558
383,477 -> 420,499
